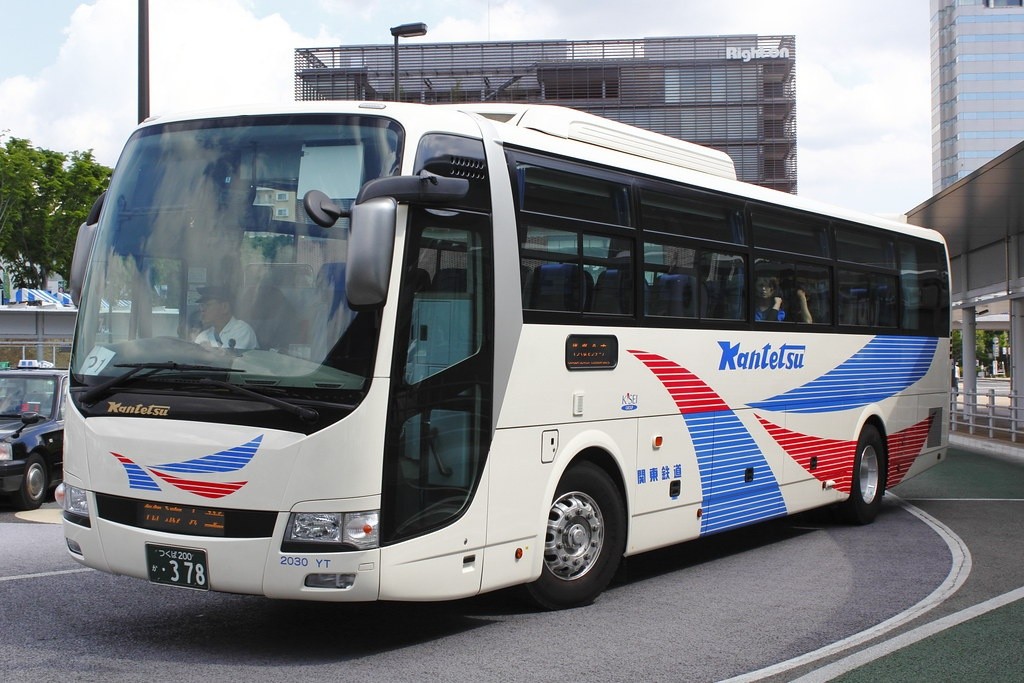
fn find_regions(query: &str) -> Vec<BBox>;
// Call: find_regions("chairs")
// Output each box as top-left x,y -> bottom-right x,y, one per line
239,286 -> 295,351
414,261 -> 891,333
21,392 -> 48,415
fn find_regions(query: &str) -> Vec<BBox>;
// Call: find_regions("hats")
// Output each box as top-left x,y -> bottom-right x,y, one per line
194,286 -> 236,304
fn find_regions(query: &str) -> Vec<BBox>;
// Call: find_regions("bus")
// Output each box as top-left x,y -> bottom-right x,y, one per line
58,102 -> 953,610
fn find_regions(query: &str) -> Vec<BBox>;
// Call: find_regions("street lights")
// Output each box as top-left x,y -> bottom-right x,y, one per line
389,24 -> 427,102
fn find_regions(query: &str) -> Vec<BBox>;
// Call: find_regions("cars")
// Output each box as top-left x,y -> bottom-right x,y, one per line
0,358 -> 70,509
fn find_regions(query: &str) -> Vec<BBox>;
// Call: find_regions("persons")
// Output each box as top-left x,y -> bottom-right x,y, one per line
194,288 -> 261,349
754,276 -> 785,321
797,289 -> 813,323
